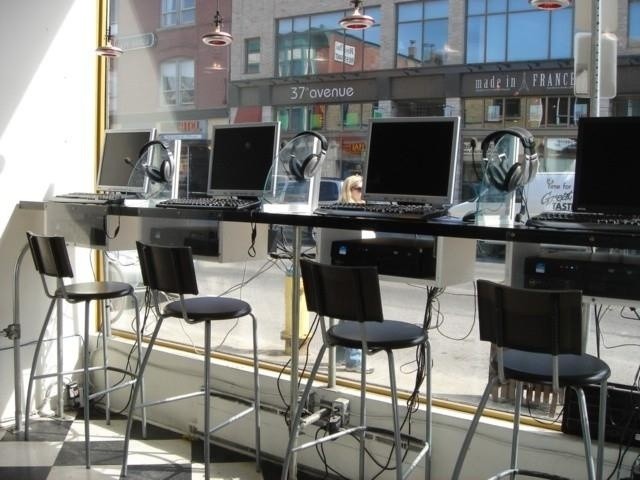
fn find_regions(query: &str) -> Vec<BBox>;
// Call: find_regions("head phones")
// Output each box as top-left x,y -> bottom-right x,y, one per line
126,139 -> 174,183
469,130 -> 533,191
290,130 -> 326,181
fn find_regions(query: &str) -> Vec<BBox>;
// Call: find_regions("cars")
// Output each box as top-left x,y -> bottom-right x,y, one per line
449,184 -> 523,224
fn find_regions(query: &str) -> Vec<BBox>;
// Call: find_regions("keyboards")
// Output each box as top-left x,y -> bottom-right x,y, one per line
527,212 -> 640,233
57,189 -> 129,206
157,198 -> 261,213
313,201 -> 447,224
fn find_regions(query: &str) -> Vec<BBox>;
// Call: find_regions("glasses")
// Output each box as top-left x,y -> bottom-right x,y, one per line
354,186 -> 361,192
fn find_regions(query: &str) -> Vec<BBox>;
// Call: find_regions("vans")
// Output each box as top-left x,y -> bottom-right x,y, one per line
268,178 -> 345,238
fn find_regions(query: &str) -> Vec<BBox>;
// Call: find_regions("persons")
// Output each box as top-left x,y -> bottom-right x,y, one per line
311,175 -> 377,374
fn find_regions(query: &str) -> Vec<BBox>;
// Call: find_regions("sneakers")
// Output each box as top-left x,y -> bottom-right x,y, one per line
345,363 -> 376,374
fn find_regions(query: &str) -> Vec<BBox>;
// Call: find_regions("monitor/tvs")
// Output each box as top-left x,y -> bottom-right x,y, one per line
205,120 -> 281,197
95,130 -> 156,193
570,117 -> 640,216
360,118 -> 461,206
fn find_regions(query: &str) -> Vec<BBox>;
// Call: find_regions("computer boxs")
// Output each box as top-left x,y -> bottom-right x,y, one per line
152,226 -> 217,257
331,237 -> 435,278
524,252 -> 640,299
46,212 -> 103,246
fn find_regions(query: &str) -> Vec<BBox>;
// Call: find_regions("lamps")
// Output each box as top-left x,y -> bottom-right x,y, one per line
529,0 -> 569,10
95,0 -> 123,57
339,0 -> 374,30
201,0 -> 232,45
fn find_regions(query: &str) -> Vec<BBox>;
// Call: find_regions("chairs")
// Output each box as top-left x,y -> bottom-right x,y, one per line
23,231 -> 147,469
450,279 -> 609,475
119,241 -> 262,475
282,260 -> 433,475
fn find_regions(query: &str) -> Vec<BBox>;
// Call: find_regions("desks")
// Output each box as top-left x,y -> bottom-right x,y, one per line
18,193 -> 639,475
